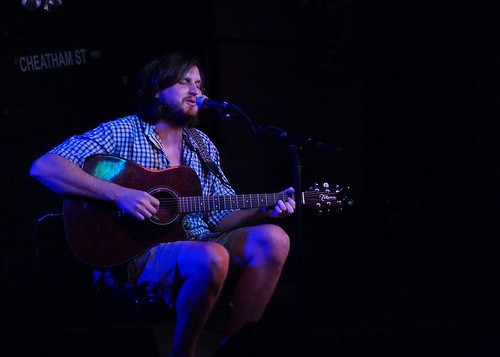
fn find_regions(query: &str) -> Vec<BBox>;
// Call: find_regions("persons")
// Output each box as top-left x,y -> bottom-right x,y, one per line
29,57 -> 297,357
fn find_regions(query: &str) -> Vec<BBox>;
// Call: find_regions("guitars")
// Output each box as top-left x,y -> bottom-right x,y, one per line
64,154 -> 353,267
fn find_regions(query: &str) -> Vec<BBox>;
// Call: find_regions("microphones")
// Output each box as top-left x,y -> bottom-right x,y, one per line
196,95 -> 237,110
